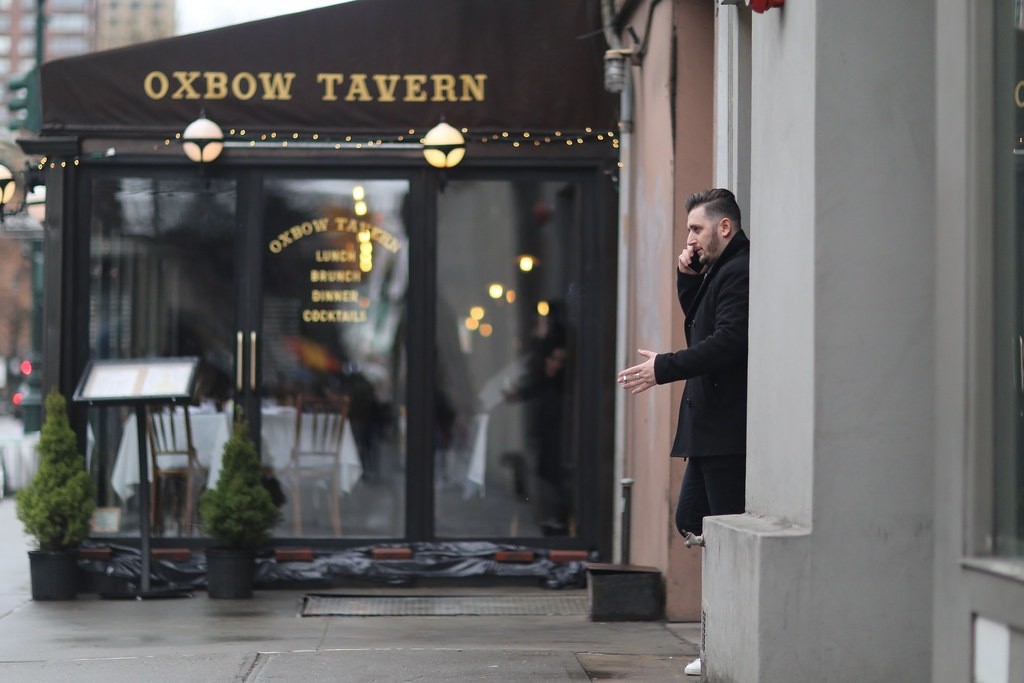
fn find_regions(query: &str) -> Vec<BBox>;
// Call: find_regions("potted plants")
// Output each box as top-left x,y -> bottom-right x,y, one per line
197,405 -> 285,601
13,385 -> 95,600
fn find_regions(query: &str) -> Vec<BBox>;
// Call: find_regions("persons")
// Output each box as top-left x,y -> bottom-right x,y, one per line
505,301 -> 568,531
619,188 -> 750,675
331,354 -> 381,490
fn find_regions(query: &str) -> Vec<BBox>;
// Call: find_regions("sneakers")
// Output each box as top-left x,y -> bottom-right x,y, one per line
684,659 -> 701,676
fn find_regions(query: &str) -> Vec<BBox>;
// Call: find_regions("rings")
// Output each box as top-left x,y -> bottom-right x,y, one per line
635,374 -> 639,379
624,375 -> 627,383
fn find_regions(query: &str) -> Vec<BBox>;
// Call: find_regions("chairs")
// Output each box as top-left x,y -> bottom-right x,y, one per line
146,402 -> 209,537
259,395 -> 353,535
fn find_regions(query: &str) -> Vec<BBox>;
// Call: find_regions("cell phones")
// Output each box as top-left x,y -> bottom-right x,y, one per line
687,252 -> 705,274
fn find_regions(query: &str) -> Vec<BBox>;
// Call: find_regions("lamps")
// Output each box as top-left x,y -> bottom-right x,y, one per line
423,121 -> 467,188
180,105 -> 225,183
0,163 -> 45,224
602,49 -> 643,93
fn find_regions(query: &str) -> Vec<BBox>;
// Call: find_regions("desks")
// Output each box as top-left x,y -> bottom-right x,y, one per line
110,414 -> 364,534
